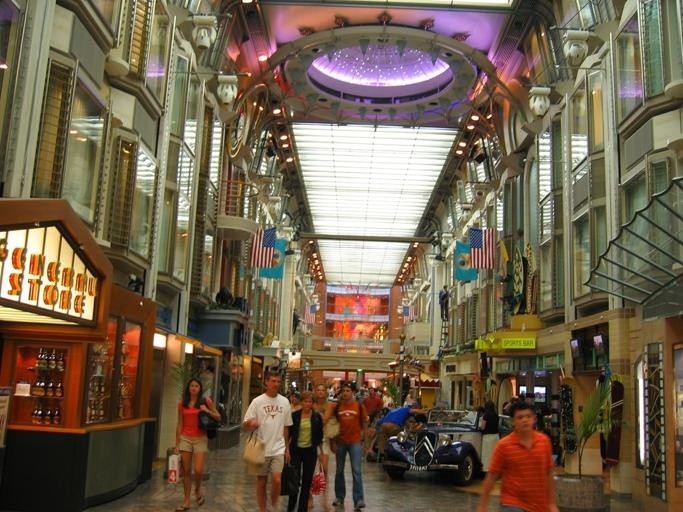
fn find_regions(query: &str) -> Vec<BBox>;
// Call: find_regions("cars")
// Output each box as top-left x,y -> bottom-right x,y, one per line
383,407 -> 511,485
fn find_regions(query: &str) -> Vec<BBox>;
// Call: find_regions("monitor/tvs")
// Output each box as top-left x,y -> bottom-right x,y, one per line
518,385 -> 547,404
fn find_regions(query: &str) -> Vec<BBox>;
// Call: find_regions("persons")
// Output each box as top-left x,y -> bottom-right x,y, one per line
240,371 -> 429,511
475,402 -> 560,511
439,285 -> 451,321
479,401 -> 500,473
173,377 -> 221,512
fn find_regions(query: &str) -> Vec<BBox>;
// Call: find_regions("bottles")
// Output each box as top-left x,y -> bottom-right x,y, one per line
85,343 -> 126,423
29,345 -> 66,425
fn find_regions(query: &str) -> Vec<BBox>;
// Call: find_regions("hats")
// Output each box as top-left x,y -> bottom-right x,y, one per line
343,381 -> 356,392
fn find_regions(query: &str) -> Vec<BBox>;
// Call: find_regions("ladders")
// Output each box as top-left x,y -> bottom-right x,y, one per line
438,298 -> 449,350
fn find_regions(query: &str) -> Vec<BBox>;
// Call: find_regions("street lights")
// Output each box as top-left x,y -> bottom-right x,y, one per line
397,333 -> 406,407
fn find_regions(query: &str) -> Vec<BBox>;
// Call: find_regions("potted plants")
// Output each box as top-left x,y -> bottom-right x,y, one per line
173,343 -> 213,479
552,380 -> 615,512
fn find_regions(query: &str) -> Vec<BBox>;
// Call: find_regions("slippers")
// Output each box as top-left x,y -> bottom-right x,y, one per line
196,494 -> 205,506
176,505 -> 191,512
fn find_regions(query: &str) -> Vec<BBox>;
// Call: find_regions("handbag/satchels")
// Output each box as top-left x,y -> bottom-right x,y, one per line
197,401 -> 221,431
332,497 -> 345,507
242,427 -> 267,466
309,462 -> 328,496
167,452 -> 184,485
354,498 -> 366,511
280,460 -> 300,496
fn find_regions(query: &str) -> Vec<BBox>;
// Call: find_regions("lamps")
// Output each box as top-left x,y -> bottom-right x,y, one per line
562,28 -> 591,66
189,14 -> 217,49
215,74 -> 239,104
528,87 -> 552,119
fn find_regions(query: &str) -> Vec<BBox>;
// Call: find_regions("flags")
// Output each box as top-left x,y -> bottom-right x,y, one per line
250,227 -> 277,269
303,304 -> 315,324
258,239 -> 287,280
403,305 -> 414,326
469,227 -> 495,271
452,240 -> 478,282
